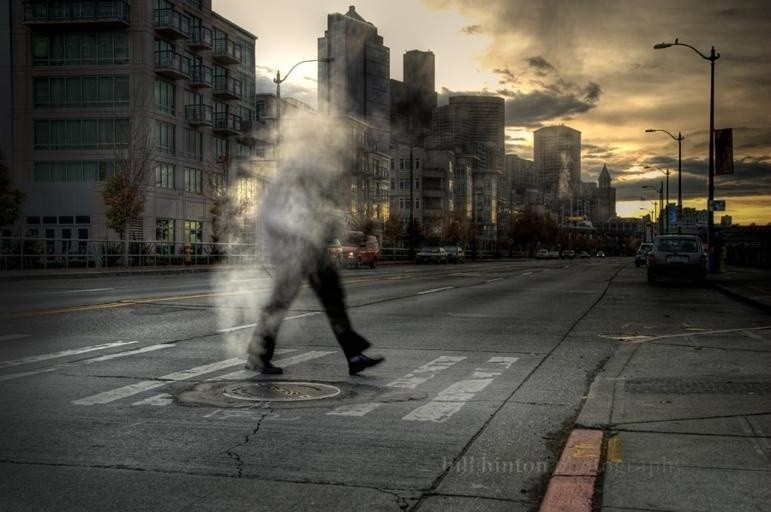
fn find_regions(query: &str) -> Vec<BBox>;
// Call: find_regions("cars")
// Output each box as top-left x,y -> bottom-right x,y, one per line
415,245 -> 465,264
635,234 -> 710,287
536,249 -> 605,259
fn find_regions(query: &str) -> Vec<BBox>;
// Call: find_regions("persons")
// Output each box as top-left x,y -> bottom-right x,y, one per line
240,203 -> 386,375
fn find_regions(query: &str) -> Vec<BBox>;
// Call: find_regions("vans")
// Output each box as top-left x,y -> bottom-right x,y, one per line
329,230 -> 380,268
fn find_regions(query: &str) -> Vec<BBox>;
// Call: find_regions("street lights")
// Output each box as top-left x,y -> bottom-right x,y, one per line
269,58 -> 334,158
654,37 -> 720,246
642,129 -> 684,233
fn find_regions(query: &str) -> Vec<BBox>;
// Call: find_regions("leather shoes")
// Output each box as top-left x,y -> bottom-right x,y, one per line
346,355 -> 386,376
244,355 -> 283,374
